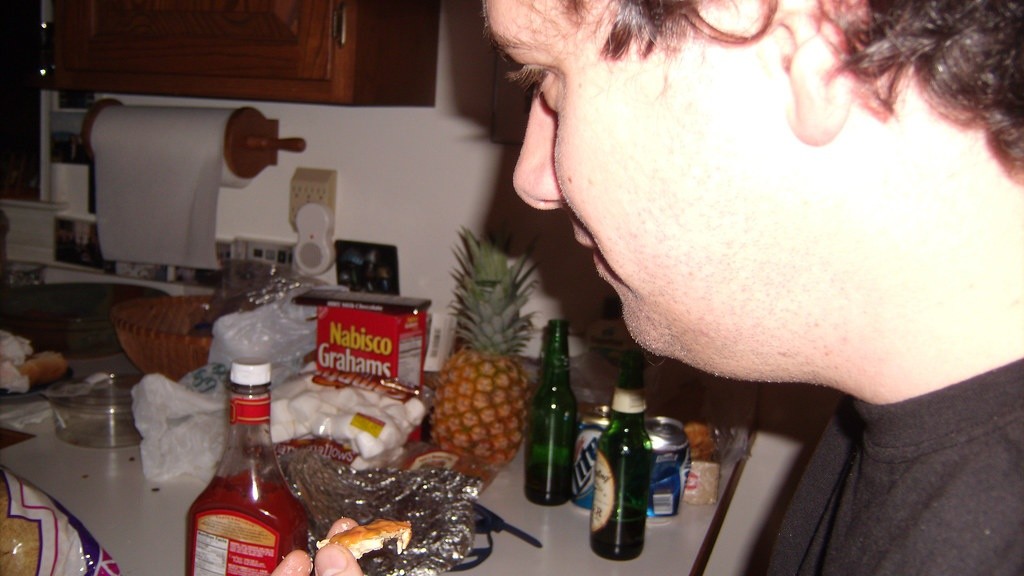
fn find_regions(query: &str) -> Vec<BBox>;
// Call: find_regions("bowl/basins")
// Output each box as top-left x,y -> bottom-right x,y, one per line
48,373 -> 144,447
110,296 -> 227,383
0,283 -> 173,361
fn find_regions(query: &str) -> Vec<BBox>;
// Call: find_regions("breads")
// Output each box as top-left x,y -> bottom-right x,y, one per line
654,378 -> 716,461
0,475 -> 42,576
14,349 -> 67,387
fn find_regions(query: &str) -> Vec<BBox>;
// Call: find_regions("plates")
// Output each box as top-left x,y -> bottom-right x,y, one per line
0,367 -> 74,400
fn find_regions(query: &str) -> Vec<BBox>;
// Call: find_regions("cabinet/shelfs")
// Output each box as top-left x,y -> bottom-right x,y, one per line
53,0 -> 442,108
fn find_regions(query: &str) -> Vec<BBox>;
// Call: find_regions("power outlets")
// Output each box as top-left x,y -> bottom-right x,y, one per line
289,169 -> 336,231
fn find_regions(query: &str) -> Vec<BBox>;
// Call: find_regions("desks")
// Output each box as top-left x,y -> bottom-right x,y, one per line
0,254 -> 772,576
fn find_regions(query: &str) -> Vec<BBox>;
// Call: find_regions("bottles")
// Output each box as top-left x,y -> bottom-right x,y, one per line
589,350 -> 653,561
524,319 -> 578,505
186,358 -> 310,576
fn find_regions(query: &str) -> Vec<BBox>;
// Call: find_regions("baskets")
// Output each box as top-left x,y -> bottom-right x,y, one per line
110,295 -> 212,383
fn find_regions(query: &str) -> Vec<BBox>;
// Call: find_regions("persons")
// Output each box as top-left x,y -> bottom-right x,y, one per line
270,0 -> 1024,576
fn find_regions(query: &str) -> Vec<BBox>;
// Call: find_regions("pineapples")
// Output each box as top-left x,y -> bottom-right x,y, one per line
429,227 -> 541,479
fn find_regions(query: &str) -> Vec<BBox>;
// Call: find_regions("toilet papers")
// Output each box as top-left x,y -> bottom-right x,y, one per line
87,103 -> 253,270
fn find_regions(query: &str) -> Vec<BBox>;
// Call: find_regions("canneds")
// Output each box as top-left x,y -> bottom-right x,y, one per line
642,415 -> 692,518
568,404 -> 614,512
